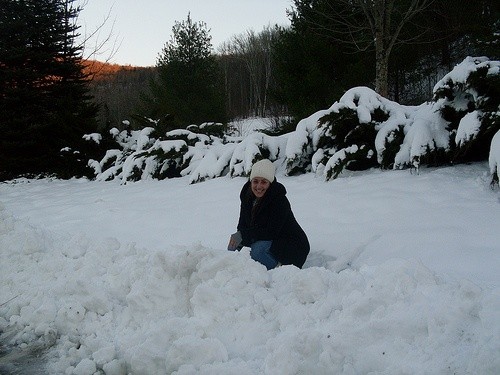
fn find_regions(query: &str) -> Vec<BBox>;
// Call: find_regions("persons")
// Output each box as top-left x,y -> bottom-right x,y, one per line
228,159 -> 310,270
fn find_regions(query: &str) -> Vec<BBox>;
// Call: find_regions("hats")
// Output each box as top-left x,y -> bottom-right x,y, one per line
250,159 -> 275,183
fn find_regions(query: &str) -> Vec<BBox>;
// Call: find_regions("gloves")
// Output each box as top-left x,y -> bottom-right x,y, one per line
228,231 -> 243,252
